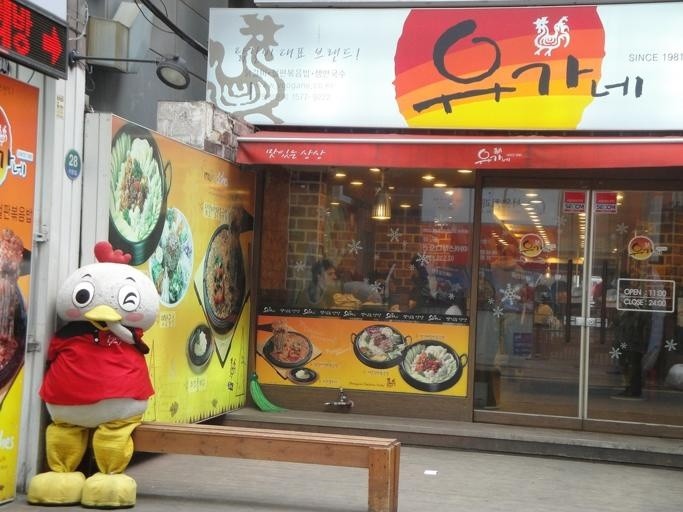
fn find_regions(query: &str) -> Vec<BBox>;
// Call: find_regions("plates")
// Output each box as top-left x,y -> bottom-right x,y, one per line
188,325 -> 214,366
289,368 -> 315,383
262,330 -> 312,370
0,245 -> 30,278
148,208 -> 194,307
200,222 -> 245,334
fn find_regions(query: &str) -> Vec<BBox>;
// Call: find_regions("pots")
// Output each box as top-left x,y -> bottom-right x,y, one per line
110,120 -> 175,267
349,324 -> 413,370
397,334 -> 469,391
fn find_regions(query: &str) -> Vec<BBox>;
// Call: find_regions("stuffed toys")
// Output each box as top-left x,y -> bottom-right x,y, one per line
23,240 -> 159,511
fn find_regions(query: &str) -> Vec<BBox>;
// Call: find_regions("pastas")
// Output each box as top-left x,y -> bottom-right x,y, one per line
109,131 -> 163,245
403,344 -> 457,384
207,219 -> 242,318
268,322 -> 305,361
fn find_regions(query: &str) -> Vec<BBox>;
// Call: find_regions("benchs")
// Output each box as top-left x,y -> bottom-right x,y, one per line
86,421 -> 402,512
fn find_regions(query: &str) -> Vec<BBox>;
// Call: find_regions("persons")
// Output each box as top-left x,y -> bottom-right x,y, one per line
295,257 -> 342,314
403,253 -> 445,317
487,242 -> 529,384
613,270 -> 654,399
514,278 -> 535,303
525,275 -> 555,360
